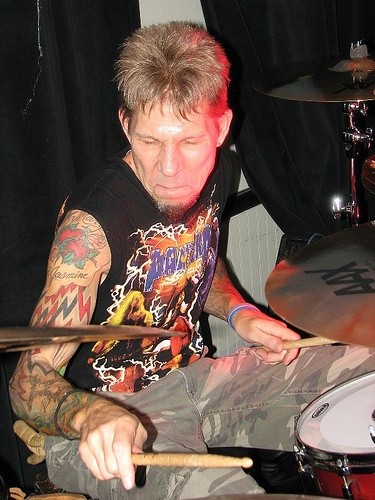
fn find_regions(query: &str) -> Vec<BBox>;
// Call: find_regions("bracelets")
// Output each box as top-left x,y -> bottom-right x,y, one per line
54,388 -> 87,441
227,303 -> 261,326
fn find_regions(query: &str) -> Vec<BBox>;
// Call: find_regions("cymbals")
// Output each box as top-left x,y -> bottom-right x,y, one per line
265,219 -> 375,349
1,323 -> 189,352
252,53 -> 375,102
360,155 -> 375,195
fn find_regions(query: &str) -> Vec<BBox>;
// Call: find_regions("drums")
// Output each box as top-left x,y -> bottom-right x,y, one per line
292,369 -> 375,500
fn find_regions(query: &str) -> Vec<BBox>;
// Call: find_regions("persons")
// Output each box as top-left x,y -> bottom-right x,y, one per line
9,21 -> 375,500
